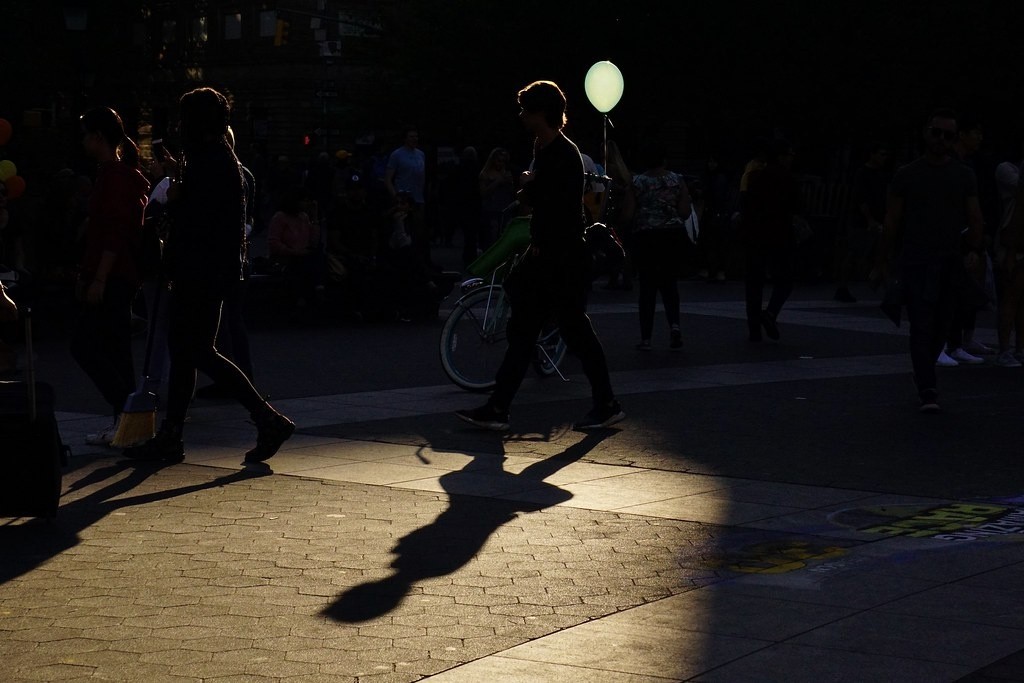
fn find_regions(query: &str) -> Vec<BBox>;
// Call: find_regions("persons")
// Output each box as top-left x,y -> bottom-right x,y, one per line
120,85 -> 299,467
453,79 -> 630,436
69,105 -> 151,450
0,105 -> 1024,415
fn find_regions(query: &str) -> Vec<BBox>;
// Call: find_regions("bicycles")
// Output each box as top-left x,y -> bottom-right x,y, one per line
439,236 -> 572,395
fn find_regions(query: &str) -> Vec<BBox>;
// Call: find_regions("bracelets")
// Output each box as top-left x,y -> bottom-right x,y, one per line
967,241 -> 978,255
94,276 -> 106,284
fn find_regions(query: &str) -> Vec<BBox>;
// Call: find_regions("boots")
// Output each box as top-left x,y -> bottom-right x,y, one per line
122,419 -> 186,460
245,395 -> 296,462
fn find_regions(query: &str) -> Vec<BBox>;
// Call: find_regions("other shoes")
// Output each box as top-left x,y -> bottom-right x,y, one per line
749,336 -> 763,342
758,310 -> 780,340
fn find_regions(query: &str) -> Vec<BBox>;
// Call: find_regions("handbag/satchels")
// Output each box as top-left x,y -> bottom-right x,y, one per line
977,249 -> 997,314
883,280 -> 904,330
583,222 -> 624,276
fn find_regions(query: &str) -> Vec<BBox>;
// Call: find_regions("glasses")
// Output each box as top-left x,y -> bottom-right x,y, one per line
80,131 -> 88,140
927,126 -> 957,142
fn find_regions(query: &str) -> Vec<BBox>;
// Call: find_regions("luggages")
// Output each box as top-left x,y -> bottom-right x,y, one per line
0,303 -> 72,519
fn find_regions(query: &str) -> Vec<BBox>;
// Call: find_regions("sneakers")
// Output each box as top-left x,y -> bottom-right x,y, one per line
455,401 -> 510,431
671,325 -> 681,343
636,339 -> 652,350
964,340 -> 995,355
573,400 -> 626,430
935,350 -> 958,367
87,425 -> 115,445
946,351 -> 985,365
912,374 -> 942,412
993,352 -> 1024,367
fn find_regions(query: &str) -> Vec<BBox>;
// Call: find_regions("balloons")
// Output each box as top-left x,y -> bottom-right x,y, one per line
584,60 -> 627,117
